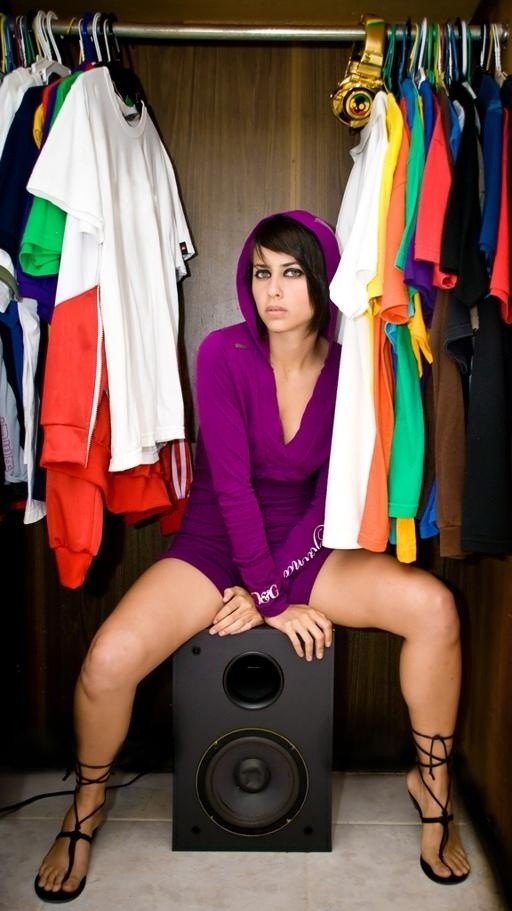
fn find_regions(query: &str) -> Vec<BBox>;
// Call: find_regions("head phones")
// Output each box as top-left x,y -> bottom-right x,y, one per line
325,13 -> 395,134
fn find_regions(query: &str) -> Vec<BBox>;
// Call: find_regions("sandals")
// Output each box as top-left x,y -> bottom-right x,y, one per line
403,728 -> 472,885
33,754 -> 115,905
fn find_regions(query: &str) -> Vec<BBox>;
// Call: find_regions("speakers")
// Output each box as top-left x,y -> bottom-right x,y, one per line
170,621 -> 337,855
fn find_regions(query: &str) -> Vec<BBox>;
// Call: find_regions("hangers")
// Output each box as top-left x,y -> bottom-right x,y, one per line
379,16 -> 511,99
1,9 -> 145,117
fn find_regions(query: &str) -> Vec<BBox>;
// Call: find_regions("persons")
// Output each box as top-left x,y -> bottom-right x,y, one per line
32,210 -> 473,905
